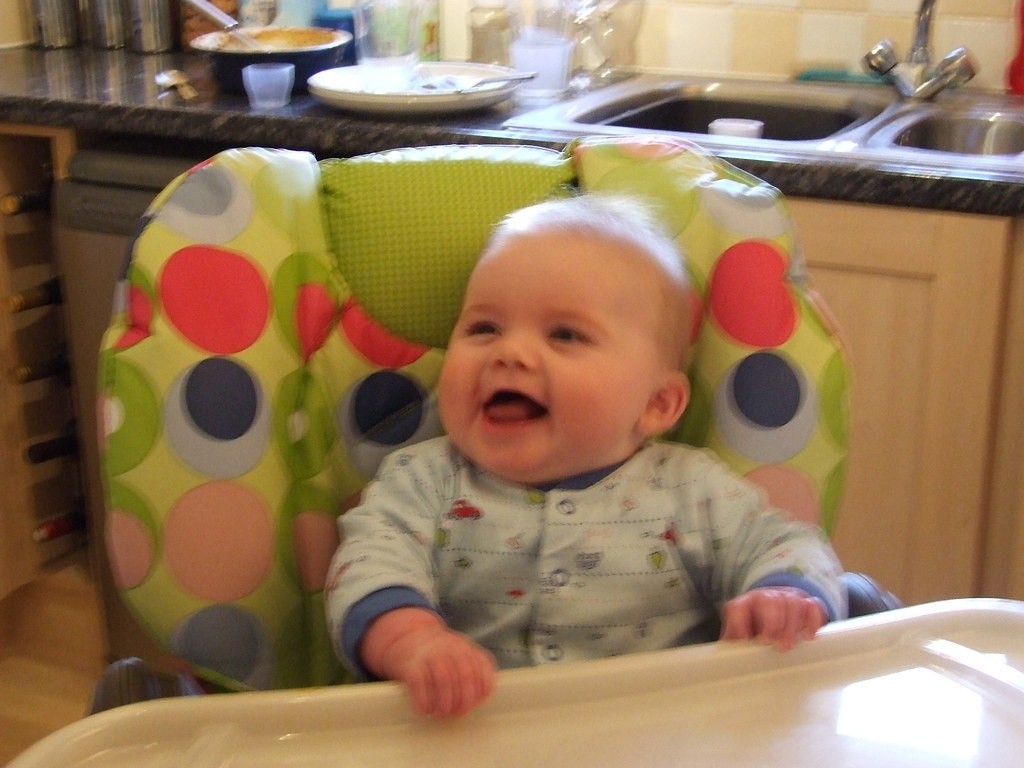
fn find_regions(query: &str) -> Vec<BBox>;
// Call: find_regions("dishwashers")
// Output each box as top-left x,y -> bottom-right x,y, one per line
48,149 -> 199,679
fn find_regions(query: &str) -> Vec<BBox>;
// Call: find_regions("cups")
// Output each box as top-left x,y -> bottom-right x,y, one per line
350,0 -> 420,90
509,38 -> 572,108
240,64 -> 294,110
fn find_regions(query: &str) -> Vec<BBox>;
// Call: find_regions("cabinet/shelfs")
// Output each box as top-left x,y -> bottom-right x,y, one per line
775,195 -> 1017,611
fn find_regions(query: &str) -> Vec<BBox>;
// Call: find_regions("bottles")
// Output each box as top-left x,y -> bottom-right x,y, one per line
464,0 -> 515,67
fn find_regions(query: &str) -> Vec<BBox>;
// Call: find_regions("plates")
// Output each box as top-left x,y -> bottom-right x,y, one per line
306,62 -> 526,115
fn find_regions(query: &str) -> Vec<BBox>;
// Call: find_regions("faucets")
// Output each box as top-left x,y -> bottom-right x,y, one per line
860,1 -> 979,103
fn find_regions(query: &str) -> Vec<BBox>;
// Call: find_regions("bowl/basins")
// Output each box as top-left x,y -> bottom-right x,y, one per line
189,26 -> 353,95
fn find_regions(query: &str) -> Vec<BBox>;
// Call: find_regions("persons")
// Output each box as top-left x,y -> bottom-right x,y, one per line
322,193 -> 853,715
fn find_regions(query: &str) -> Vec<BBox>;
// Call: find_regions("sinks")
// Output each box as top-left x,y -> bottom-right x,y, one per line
861,87 -> 1024,183
502,73 -> 897,161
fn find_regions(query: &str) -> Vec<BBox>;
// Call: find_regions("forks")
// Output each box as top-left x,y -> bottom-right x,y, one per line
421,71 -> 540,90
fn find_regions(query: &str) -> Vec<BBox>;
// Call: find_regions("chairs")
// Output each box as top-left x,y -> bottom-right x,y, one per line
85,132 -> 906,717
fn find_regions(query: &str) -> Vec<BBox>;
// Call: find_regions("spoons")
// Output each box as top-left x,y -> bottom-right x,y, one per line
154,69 -> 199,100
